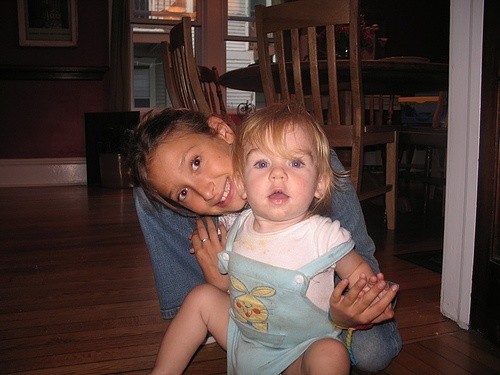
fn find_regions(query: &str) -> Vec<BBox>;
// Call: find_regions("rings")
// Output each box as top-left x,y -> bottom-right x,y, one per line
201,238 -> 209,243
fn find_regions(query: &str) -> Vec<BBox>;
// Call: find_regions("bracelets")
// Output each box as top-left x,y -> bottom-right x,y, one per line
328,308 -> 358,367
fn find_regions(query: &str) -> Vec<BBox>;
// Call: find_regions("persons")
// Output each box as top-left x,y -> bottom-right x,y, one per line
151,102 -> 391,375
122,107 -> 402,373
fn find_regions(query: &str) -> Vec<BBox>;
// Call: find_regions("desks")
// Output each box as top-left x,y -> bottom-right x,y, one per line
218,60 -> 449,219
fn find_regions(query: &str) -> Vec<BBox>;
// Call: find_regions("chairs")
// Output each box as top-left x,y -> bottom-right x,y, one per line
161,0 -> 450,231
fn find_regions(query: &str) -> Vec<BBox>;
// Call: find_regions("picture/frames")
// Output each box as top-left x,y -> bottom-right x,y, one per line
17,0 -> 78,47
